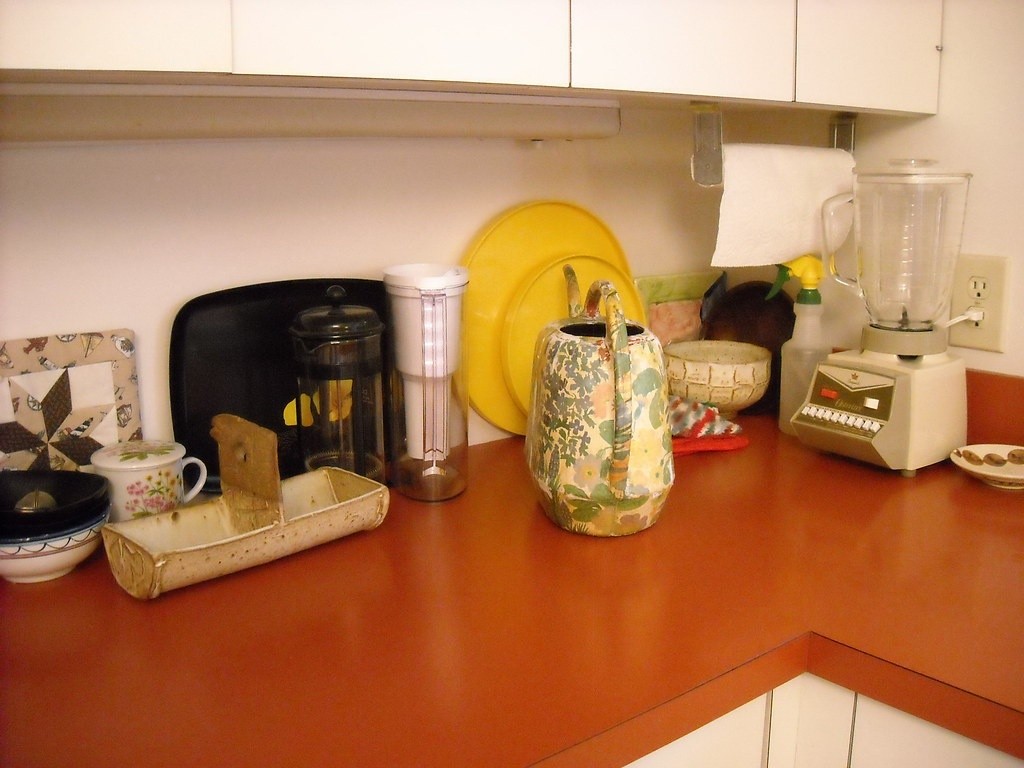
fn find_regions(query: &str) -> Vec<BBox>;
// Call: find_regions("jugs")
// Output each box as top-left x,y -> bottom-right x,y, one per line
383,263 -> 469,501
523,265 -> 674,536
289,286 -> 384,485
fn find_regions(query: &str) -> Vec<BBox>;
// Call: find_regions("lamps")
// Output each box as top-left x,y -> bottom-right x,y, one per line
0,83 -> 619,142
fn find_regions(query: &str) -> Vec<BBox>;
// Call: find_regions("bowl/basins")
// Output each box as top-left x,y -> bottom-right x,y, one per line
0,470 -> 111,584
662,340 -> 772,420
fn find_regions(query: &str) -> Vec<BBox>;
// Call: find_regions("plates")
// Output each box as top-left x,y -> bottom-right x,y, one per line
698,281 -> 796,415
950,444 -> 1024,490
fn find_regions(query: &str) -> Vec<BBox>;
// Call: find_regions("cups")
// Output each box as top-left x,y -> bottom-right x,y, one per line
91,440 -> 207,522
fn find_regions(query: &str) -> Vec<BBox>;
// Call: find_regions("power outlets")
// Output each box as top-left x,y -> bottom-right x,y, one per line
949,254 -> 1008,352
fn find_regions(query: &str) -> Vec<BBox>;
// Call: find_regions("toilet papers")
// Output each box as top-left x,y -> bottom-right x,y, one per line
691,142 -> 857,267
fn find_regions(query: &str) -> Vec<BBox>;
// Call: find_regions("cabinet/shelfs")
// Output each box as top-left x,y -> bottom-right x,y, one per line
620,672 -> 1023,768
0,2 -> 941,118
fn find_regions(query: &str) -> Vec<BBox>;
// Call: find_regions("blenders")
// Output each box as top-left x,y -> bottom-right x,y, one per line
790,158 -> 973,477
